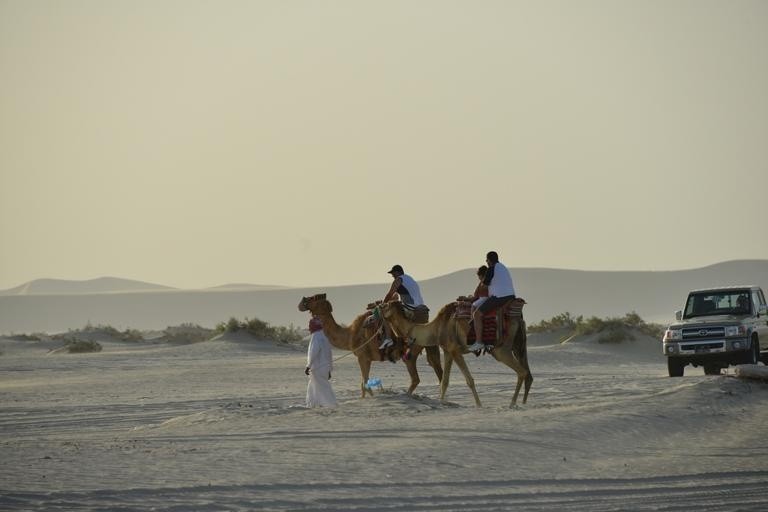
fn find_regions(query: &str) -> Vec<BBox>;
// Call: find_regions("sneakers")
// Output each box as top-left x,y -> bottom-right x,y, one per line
469,344 -> 484,352
378,339 -> 393,349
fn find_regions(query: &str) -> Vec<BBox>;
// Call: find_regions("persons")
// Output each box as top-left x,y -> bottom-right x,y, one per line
305,316 -> 340,408
466,251 -> 517,352
376,264 -> 424,351
455,264 -> 489,325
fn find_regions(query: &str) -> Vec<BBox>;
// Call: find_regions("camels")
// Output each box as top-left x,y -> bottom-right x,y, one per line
378,296 -> 534,410
297,292 -> 444,399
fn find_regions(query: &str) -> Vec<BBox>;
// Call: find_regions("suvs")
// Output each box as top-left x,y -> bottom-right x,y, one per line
659,283 -> 767,378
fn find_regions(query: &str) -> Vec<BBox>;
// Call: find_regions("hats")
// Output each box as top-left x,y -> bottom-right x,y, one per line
387,265 -> 404,274
